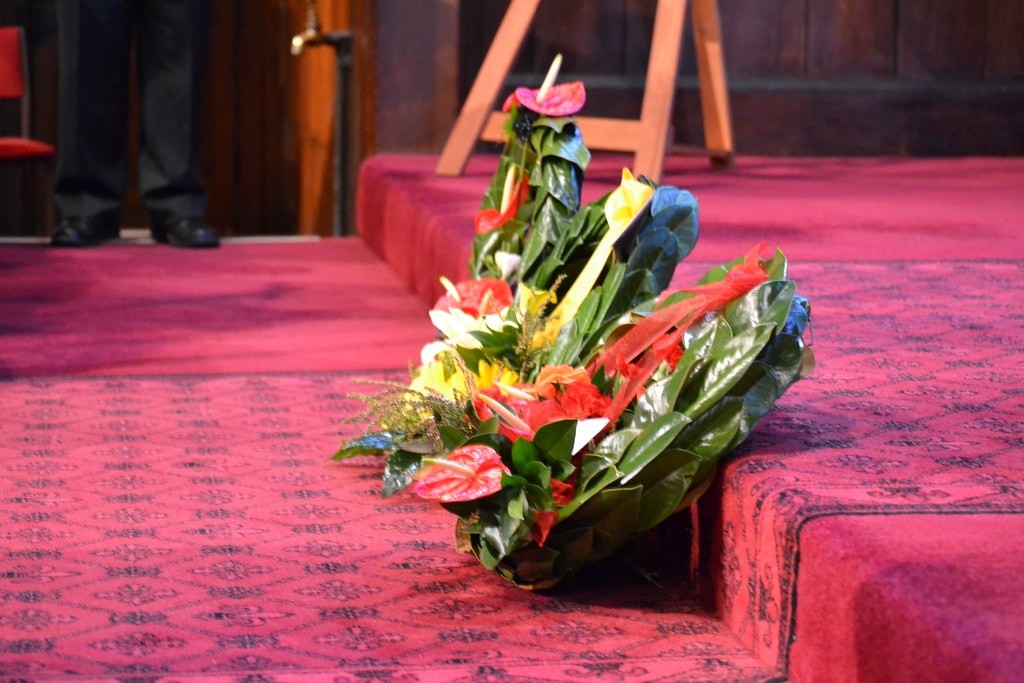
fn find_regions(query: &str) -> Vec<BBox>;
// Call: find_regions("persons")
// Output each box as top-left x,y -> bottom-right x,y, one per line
50,0 -> 219,247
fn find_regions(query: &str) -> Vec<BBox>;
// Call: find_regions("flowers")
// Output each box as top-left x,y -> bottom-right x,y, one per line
330,53 -> 816,588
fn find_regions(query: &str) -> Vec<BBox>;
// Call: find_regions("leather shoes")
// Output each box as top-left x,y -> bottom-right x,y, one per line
150,217 -> 218,248
50,224 -> 119,247
